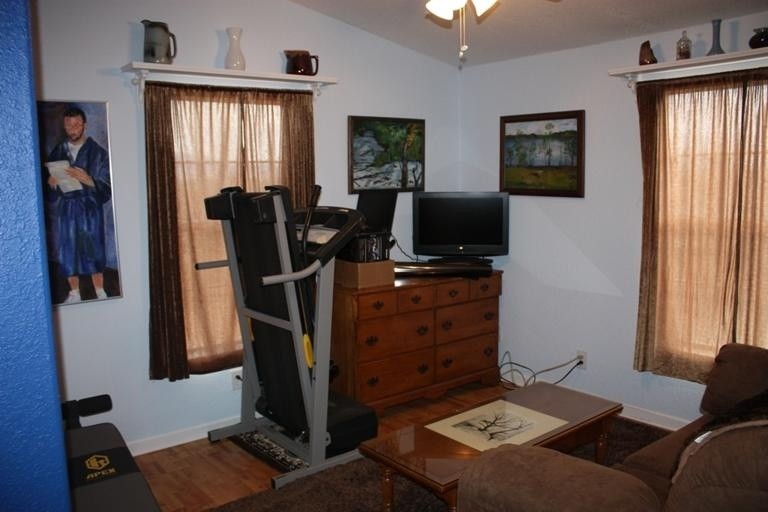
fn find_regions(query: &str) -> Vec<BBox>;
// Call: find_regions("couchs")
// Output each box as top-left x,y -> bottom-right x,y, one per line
458,341 -> 768,512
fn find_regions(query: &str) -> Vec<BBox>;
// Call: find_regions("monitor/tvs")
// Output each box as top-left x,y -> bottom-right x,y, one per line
413,191 -> 509,267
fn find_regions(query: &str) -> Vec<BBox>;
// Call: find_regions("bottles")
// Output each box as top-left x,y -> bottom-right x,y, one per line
676,29 -> 692,60
226,26 -> 246,71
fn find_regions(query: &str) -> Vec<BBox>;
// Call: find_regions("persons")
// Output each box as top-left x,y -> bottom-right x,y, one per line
44,105 -> 112,305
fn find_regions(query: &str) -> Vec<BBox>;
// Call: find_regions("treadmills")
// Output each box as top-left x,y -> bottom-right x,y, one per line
195,185 -> 378,490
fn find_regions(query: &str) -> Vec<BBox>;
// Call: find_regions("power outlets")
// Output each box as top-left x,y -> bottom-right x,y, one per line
575,349 -> 587,368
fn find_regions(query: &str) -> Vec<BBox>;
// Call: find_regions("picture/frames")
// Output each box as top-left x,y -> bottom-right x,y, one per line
347,115 -> 426,195
35,97 -> 124,308
500,110 -> 585,199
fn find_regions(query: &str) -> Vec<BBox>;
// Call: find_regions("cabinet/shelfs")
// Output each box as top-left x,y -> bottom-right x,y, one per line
314,271 -> 504,419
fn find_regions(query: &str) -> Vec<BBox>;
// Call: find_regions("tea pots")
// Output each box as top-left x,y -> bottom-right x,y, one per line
284,51 -> 319,76
140,19 -> 177,65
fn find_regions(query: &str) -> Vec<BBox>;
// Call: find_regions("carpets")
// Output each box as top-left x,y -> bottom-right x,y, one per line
203,415 -> 671,511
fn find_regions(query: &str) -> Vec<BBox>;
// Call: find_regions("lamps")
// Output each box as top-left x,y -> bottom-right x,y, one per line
425,1 -> 497,61
